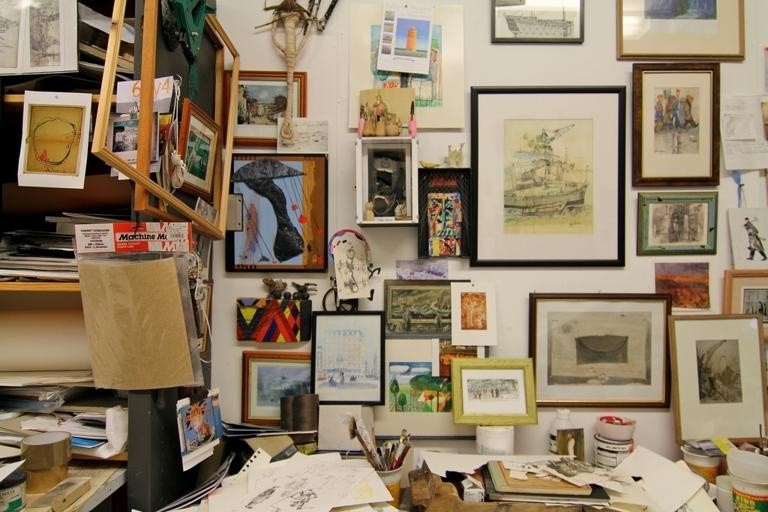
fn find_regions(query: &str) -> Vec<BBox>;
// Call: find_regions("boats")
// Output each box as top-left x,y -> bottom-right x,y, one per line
493,7 -> 578,39
504,123 -> 588,220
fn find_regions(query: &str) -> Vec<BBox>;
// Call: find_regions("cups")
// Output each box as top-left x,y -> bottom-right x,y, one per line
376,464 -> 402,507
382,438 -> 413,488
679,444 -> 727,485
279,394 -> 320,430
714,474 -> 734,511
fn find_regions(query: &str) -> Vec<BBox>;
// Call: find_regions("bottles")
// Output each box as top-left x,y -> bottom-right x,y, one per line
548,406 -> 573,457
372,95 -> 388,136
406,26 -> 417,52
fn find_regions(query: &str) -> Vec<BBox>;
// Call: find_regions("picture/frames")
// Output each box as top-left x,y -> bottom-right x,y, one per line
447,357 -> 539,426
636,188 -> 718,256
631,62 -> 721,187
240,350 -> 312,428
466,84 -> 630,270
309,311 -> 387,408
667,310 -> 768,446
178,97 -> 223,200
490,1 -> 587,45
226,153 -> 331,274
382,279 -> 472,336
372,334 -> 490,439
723,268 -> 767,368
222,69 -> 307,148
87,1 -> 242,242
527,292 -> 675,410
450,280 -> 500,348
615,0 -> 750,62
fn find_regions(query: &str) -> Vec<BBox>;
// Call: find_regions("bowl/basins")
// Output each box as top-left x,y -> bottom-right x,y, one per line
597,412 -> 635,441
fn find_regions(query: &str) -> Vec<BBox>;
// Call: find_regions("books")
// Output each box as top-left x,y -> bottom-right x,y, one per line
487,458 -> 592,495
483,465 -> 611,503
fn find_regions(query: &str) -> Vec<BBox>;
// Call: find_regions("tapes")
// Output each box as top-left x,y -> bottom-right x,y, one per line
21,430 -> 73,494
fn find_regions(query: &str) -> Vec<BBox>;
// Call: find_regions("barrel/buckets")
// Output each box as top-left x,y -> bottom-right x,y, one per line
680,445 -> 720,483
729,471 -> 768,512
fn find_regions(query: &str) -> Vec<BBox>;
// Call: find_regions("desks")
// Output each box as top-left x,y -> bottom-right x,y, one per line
23,459 -> 128,511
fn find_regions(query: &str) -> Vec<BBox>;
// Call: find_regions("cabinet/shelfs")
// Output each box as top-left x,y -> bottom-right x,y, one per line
0,80 -> 136,293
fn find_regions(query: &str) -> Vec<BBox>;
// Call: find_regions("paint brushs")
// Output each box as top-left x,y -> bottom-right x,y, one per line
349,414 -> 411,471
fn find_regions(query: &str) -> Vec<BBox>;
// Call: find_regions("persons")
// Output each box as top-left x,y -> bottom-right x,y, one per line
744,217 -> 767,261
567,433 -> 577,459
659,214 -> 685,244
653,89 -> 698,153
240,177 -> 305,265
